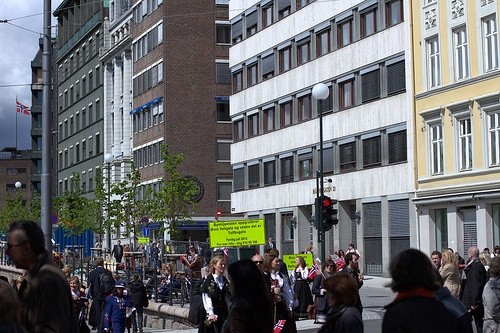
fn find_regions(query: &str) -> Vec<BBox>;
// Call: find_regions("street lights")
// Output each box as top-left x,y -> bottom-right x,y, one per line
311,82 -> 330,279
103,153 -> 113,254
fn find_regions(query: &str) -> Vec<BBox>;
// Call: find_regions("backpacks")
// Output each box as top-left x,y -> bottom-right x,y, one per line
94,268 -> 115,293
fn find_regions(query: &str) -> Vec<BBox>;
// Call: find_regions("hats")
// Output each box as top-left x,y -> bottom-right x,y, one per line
115,279 -> 127,288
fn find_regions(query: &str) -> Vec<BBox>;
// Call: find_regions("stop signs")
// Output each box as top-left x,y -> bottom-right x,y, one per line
217,209 -> 222,216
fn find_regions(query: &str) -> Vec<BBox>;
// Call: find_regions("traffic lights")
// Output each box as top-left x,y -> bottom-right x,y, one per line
329,198 -> 338,229
320,195 -> 331,232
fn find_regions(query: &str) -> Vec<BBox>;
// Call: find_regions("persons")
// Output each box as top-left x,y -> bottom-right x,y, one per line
381,248 -> 475,333
314,273 -> 365,333
429,245 -> 500,333
0,218 -> 363,333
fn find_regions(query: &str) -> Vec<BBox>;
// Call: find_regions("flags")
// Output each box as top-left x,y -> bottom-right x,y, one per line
15,100 -> 30,115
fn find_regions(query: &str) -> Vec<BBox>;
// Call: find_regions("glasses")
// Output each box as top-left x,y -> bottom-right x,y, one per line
325,264 -> 334,268
7,241 -> 30,249
254,260 -> 264,265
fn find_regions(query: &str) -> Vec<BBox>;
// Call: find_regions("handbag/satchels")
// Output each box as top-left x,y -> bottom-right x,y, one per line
188,293 -> 206,325
